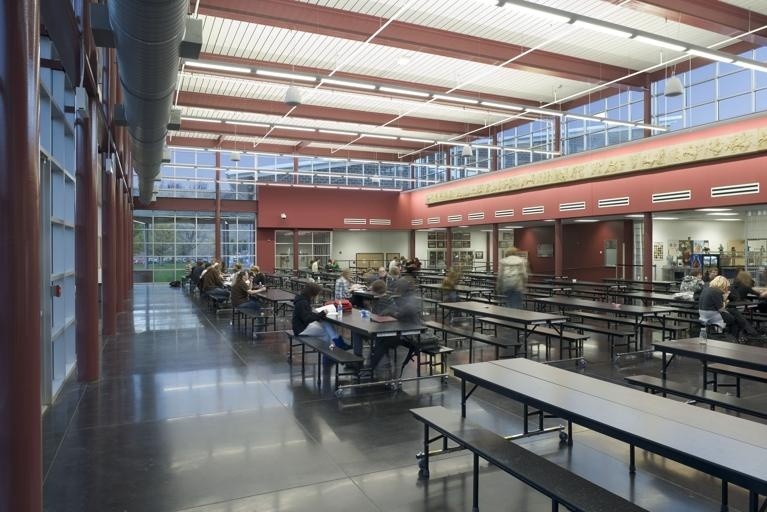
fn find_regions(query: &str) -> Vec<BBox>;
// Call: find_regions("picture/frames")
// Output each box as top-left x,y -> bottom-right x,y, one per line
426,231 -> 483,266
497,231 -> 513,249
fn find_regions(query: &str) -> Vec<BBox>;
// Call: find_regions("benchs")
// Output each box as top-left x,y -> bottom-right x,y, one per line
181,266 -> 765,418
400,402 -> 650,511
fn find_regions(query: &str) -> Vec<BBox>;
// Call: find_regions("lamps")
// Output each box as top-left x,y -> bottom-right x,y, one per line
158,0 -> 766,193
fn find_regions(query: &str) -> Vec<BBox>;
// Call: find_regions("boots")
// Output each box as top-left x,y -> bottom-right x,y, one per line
332,335 -> 352,350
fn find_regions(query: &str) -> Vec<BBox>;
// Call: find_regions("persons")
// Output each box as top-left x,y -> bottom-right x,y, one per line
692,266 -> 719,301
698,274 -> 759,342
494,246 -> 529,309
439,262 -> 461,316
679,268 -> 704,300
730,270 -> 766,305
186,254 -> 422,377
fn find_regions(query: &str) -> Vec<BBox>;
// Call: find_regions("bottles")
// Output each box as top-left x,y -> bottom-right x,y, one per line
337,301 -> 342,312
698,328 -> 706,344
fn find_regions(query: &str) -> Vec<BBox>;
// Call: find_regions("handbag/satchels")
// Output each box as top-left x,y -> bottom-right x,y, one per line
324,300 -> 353,314
410,333 -> 440,352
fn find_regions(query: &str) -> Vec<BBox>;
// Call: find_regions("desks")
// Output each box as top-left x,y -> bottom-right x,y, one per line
447,354 -> 766,496
